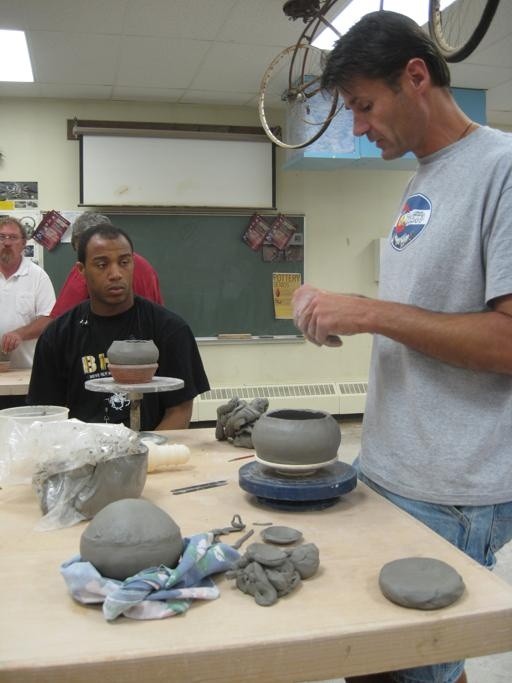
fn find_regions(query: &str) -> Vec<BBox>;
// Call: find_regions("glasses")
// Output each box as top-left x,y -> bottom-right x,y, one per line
0,234 -> 23,241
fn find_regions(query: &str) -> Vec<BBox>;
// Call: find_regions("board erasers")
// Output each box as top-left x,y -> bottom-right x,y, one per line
218,334 -> 253,339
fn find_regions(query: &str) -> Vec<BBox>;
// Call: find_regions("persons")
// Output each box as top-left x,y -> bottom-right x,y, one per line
290,10 -> 512,683
47,211 -> 164,320
25,224 -> 210,431
0,216 -> 58,367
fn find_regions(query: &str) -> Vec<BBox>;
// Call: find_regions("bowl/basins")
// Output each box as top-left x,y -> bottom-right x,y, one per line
0,360 -> 12,373
106,363 -> 159,384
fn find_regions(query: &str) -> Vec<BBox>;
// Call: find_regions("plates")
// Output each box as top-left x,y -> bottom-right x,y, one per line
254,455 -> 341,477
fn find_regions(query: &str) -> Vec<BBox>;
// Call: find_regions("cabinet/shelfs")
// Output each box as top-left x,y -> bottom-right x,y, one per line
280,75 -> 360,171
353,86 -> 488,170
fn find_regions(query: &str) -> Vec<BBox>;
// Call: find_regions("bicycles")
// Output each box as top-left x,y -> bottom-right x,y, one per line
256,0 -> 501,150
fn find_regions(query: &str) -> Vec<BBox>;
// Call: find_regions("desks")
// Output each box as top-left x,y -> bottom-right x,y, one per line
0,368 -> 32,396
0,426 -> 512,683
85,376 -> 185,431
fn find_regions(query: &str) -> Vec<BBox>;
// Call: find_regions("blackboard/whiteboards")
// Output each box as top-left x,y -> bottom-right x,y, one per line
42,208 -> 305,345
